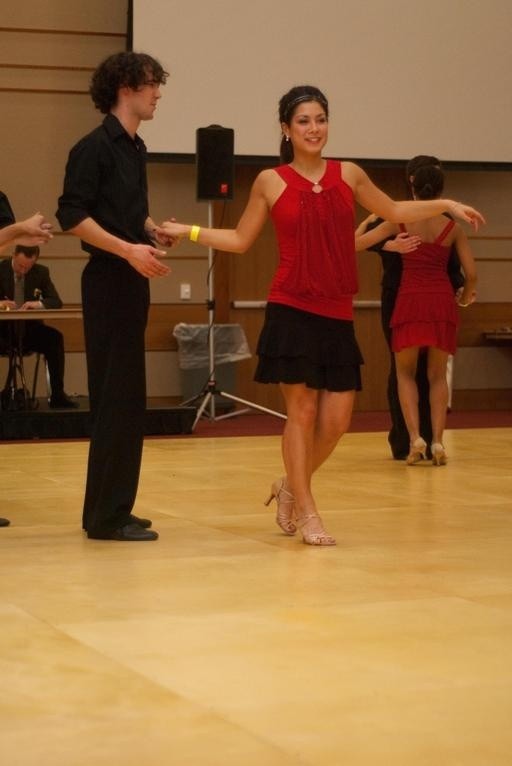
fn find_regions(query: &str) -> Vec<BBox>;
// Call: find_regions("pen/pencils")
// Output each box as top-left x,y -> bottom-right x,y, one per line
5,295 -> 18,306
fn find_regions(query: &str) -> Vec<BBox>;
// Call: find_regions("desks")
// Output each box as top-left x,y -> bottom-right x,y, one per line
0,304 -> 85,323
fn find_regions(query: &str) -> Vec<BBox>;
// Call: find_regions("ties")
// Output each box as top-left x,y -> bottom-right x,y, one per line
16,274 -> 22,281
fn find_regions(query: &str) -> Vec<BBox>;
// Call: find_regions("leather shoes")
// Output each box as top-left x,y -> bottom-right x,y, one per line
83,513 -> 152,532
49,396 -> 80,408
87,523 -> 159,541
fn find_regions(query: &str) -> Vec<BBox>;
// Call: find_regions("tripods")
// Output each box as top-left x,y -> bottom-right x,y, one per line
173,214 -> 291,435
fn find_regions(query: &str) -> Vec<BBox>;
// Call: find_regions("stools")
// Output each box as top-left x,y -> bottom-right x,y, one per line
2,345 -> 52,409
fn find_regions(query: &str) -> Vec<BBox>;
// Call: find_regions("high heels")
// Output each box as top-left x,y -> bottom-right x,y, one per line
430,443 -> 447,466
293,507 -> 336,546
405,437 -> 428,465
264,477 -> 296,535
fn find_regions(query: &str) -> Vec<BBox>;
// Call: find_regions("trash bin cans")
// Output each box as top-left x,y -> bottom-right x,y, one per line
173,322 -> 242,409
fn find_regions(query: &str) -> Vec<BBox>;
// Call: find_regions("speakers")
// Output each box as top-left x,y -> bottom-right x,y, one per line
194,122 -> 237,206
